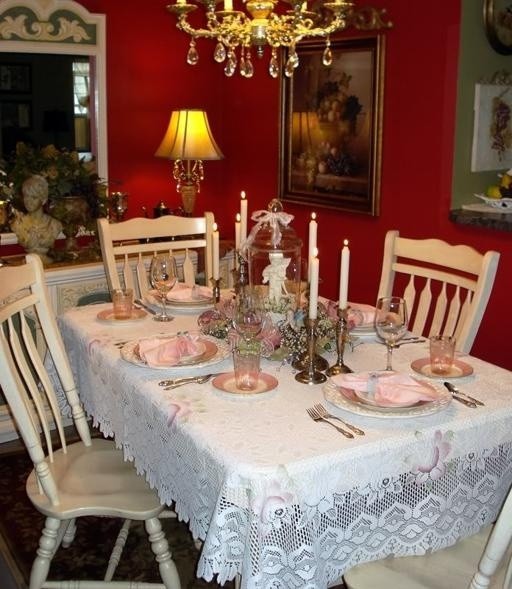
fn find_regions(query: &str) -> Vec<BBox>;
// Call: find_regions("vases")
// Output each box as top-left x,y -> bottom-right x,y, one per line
51,196 -> 89,259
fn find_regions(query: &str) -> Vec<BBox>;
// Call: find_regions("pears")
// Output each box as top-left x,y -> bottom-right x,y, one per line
502,173 -> 511,188
486,185 -> 503,199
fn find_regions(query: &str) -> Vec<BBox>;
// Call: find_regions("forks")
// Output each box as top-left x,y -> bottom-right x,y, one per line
306,402 -> 364,439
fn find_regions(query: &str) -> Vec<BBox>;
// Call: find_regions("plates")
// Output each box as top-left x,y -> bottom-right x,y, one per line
121,333 -> 232,369
97,308 -> 149,321
323,355 -> 474,417
212,370 -> 279,395
145,284 -> 225,311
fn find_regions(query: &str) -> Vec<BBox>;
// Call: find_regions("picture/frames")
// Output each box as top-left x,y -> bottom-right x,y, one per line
278,33 -> 386,216
0,100 -> 33,131
0,63 -> 32,95
482,0 -> 512,56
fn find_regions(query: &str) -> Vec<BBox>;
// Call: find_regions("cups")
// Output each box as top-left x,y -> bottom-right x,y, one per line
232,347 -> 260,391
110,289 -> 133,320
431,334 -> 455,375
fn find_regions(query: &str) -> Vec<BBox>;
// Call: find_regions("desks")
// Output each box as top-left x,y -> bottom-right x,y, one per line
56,289 -> 511,588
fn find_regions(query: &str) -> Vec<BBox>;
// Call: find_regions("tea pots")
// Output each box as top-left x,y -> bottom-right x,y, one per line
140,198 -> 175,243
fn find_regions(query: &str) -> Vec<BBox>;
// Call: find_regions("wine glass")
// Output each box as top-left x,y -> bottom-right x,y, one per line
149,255 -> 177,322
231,291 -> 268,348
373,297 -> 409,371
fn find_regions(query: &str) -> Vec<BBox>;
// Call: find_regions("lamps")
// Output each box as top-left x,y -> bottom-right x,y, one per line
166,0 -> 356,79
293,111 -> 319,155
154,110 -> 225,216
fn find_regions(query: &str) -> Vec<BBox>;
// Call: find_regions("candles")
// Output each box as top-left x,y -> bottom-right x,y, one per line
235,221 -> 242,250
309,221 -> 317,283
309,253 -> 320,320
338,246 -> 350,309
241,191 -> 247,242
211,231 -> 220,280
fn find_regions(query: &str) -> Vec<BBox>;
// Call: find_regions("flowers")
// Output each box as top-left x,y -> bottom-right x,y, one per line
16,143 -> 121,226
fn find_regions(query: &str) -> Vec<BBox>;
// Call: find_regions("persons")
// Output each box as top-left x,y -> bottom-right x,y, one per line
263,255 -> 292,306
303,91 -> 372,171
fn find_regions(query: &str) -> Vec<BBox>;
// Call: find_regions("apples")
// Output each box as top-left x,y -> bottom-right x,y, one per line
320,93 -> 347,122
331,147 -> 339,156
318,161 -> 328,173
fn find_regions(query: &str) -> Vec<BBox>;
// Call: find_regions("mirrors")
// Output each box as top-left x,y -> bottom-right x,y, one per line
0,0 -> 108,247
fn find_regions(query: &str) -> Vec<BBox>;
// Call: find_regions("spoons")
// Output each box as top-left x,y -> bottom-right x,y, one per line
394,340 -> 426,348
443,381 -> 484,407
164,374 -> 211,391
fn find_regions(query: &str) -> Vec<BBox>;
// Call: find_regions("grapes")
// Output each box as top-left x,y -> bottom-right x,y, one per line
499,185 -> 511,198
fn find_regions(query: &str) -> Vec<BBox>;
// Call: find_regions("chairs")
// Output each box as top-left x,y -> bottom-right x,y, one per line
0,253 -> 186,588
376,229 -> 501,354
342,482 -> 512,588
99,212 -> 218,299
